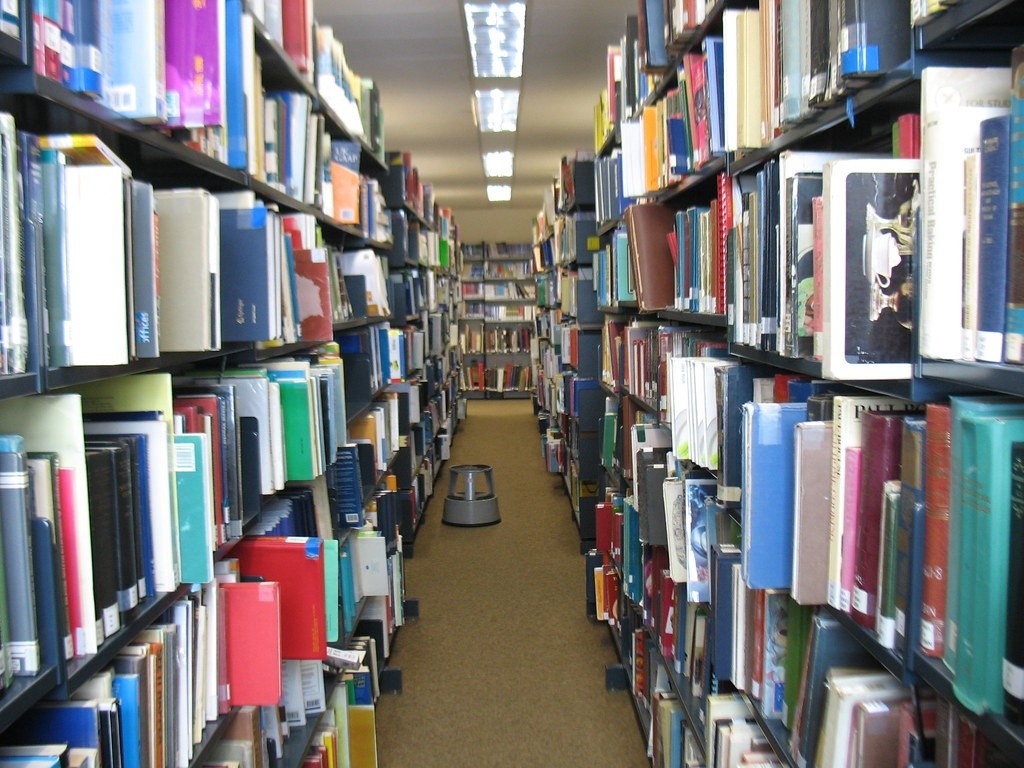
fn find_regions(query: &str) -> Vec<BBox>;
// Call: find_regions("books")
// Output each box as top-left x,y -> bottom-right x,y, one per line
0,0 -> 463,768
527,0 -> 1024,768
461,243 -> 533,390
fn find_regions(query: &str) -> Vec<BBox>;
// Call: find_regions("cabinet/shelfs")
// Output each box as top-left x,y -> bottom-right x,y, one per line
0,0 -> 1024,768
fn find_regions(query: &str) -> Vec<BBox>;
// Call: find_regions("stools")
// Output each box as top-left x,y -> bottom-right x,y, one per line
442,464 -> 500,528
